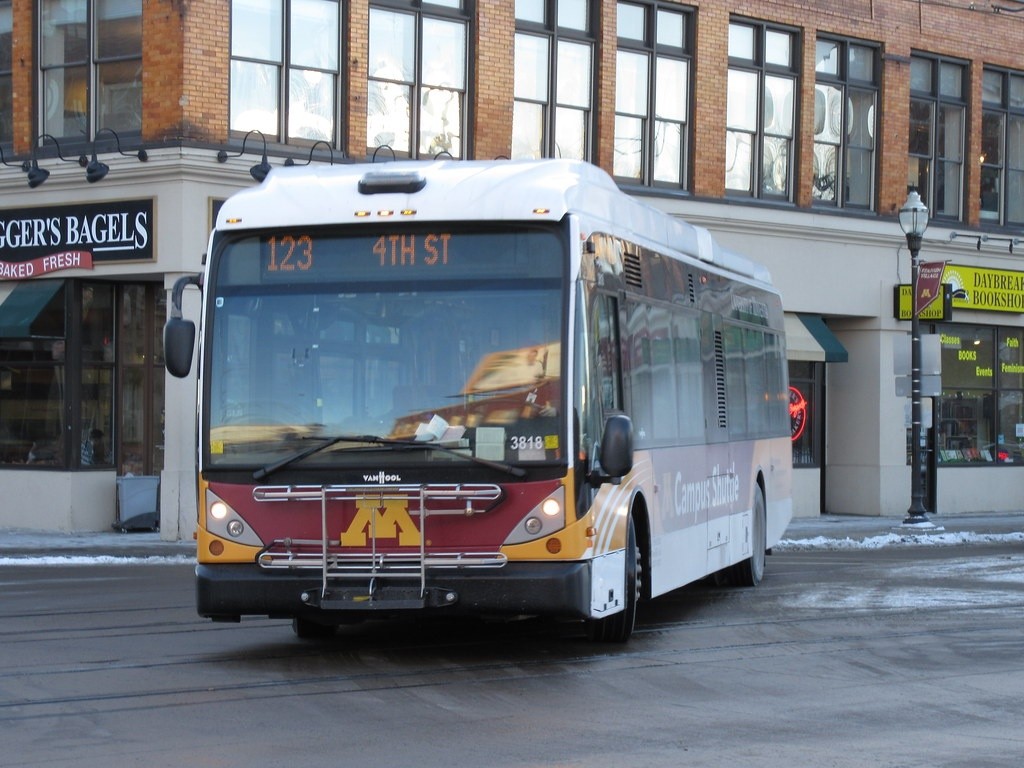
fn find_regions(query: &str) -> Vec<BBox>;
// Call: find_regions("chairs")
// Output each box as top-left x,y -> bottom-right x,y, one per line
275,357 -> 492,433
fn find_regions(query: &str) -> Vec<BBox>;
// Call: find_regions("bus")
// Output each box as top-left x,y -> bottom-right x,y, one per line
161,158 -> 799,658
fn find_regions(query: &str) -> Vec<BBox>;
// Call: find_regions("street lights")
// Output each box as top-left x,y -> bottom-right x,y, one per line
897,188 -> 935,526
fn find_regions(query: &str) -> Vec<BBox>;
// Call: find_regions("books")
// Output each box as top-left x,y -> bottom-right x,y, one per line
935,399 -> 994,462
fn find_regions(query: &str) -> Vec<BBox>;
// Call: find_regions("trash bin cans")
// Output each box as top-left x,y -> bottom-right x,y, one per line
111,474 -> 161,534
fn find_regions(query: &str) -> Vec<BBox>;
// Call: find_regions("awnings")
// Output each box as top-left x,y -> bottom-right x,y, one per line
784,311 -> 851,364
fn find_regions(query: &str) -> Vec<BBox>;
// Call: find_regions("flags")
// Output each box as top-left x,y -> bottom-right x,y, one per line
914,259 -> 947,316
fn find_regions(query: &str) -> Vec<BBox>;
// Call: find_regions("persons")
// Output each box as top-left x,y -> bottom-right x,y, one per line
465,383 -> 557,428
81,430 -> 105,467
515,348 -> 546,383
49,430 -> 76,469
